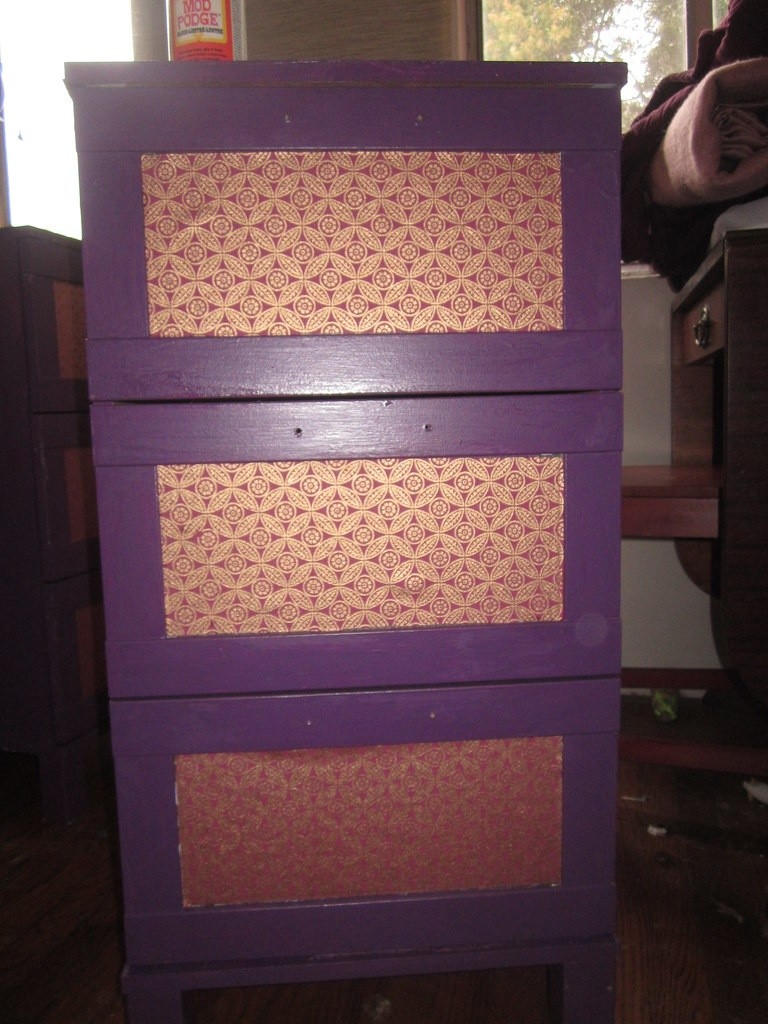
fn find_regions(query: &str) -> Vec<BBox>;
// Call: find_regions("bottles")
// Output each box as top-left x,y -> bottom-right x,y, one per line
168,0 -> 234,61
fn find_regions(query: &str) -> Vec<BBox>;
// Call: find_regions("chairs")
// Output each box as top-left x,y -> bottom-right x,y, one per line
619,464 -> 768,778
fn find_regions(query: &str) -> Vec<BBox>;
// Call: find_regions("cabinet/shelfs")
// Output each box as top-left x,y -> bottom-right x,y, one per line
0,228 -> 112,823
64,61 -> 630,1024
669,227 -> 768,709
